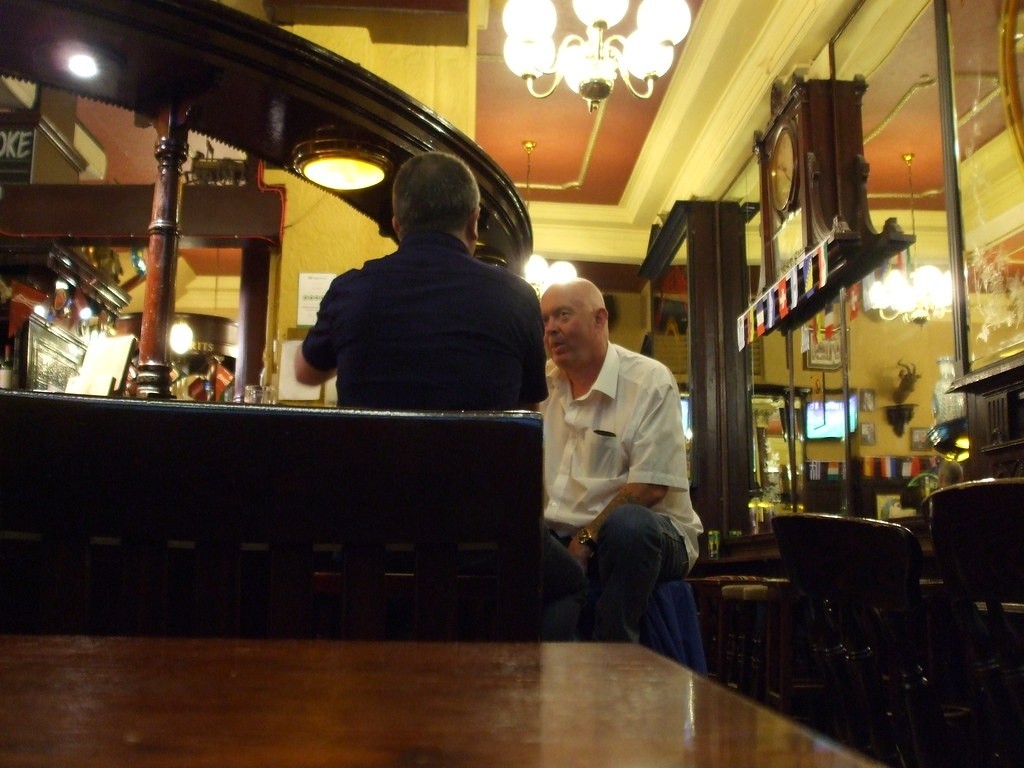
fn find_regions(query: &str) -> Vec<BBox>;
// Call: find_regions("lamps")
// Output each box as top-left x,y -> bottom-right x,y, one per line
167,313 -> 192,354
519,140 -> 578,296
869,153 -> 952,329
291,134 -> 392,192
503,0 -> 692,111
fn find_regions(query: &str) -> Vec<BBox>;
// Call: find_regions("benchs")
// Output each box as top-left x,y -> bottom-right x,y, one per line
0,389 -> 543,642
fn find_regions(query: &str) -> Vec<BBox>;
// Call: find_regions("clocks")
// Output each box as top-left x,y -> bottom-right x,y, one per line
752,73 -> 915,335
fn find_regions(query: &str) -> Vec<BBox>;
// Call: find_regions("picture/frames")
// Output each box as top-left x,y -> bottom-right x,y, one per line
860,422 -> 876,445
910,427 -> 931,450
860,389 -> 876,412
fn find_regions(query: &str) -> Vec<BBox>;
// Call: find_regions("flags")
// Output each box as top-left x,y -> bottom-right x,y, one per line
790,266 -> 798,310
747,308 -> 755,345
862,247 -> 911,311
801,320 -> 810,354
818,240 -> 828,289
816,309 -> 823,342
812,316 -> 817,345
778,278 -> 788,319
803,254 -> 814,299
766,289 -> 776,329
824,303 -> 834,340
756,299 -> 765,337
809,456 -> 921,480
849,284 -> 860,321
737,315 -> 745,352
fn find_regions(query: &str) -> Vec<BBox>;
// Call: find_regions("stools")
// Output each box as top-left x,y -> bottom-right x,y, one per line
696,574 -> 790,682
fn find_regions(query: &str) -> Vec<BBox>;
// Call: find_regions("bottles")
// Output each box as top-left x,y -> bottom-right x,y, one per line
931,356 -> 967,424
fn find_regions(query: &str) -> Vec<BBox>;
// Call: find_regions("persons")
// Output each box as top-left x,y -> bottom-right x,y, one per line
292,152 -> 549,413
537,276 -> 704,644
938,463 -> 964,489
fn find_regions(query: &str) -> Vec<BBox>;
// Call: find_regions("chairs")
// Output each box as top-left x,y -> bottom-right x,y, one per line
771,475 -> 1024,768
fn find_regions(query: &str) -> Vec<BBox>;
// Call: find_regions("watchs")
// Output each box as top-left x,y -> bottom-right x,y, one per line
576,527 -> 596,550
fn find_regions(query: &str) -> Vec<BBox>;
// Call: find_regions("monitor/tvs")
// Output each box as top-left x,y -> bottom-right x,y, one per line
804,393 -> 859,441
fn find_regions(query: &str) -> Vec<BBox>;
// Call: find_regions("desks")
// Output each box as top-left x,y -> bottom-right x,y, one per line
0,641 -> 888,768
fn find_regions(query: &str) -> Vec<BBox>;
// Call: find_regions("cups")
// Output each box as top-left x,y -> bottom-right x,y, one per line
245,385 -> 262,405
260,386 -> 278,404
728,531 -> 743,538
708,530 -> 719,559
